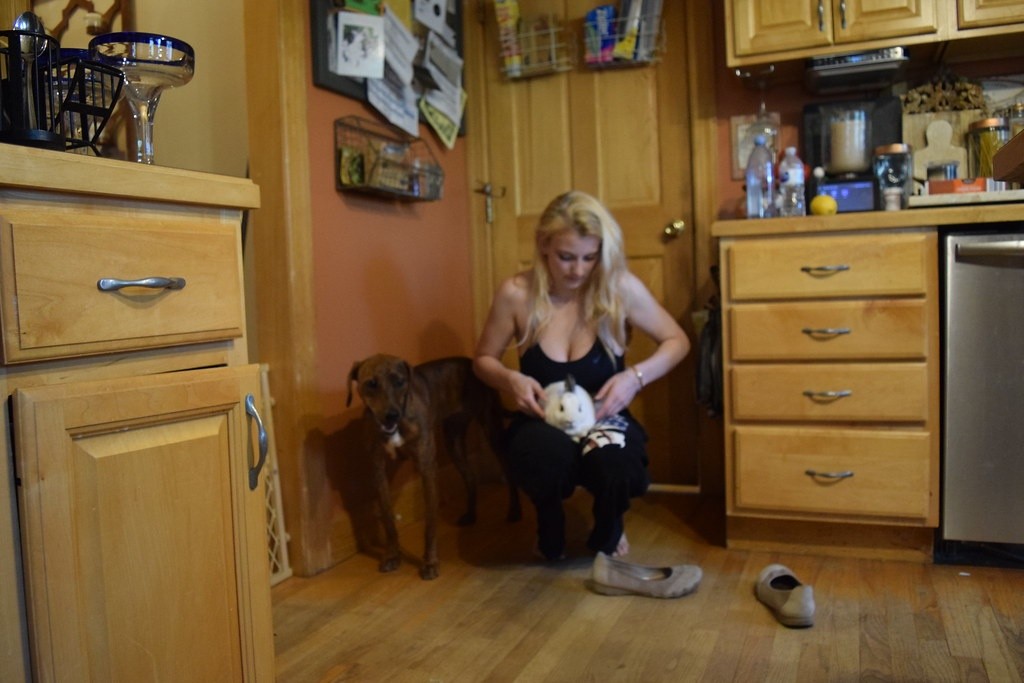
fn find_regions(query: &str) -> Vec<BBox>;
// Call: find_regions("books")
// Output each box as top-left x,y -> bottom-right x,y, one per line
494,0 -> 523,78
584,0 -> 664,64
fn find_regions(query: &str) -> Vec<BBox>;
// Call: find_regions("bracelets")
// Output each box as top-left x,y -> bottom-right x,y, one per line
633,364 -> 646,391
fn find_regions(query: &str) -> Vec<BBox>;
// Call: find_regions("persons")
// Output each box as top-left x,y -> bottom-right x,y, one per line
472,189 -> 691,560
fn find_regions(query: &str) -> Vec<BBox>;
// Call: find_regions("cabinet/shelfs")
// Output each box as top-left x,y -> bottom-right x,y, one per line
723,0 -> 1024,67
3,141 -> 279,682
718,226 -> 941,565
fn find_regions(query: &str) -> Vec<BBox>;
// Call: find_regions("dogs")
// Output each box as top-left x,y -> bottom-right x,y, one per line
346,352 -> 522,580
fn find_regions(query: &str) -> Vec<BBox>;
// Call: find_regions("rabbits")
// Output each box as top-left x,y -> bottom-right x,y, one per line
538,376 -> 597,439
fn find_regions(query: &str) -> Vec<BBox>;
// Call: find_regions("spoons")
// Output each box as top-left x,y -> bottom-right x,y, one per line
12,11 -> 47,130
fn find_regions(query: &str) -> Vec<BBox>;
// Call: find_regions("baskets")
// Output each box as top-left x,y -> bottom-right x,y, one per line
582,10 -> 668,66
496,21 -> 577,79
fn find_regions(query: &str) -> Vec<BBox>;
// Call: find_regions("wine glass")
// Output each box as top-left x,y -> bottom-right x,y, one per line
89,32 -> 195,164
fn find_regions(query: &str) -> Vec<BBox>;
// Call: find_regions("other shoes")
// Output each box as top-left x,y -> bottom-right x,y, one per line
755,564 -> 816,625
592,553 -> 704,599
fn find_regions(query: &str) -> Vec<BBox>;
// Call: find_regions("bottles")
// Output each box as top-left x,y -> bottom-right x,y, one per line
745,135 -> 774,219
778,147 -> 806,217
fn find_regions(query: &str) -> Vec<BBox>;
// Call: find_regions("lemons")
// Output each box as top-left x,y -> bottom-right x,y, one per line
809,194 -> 837,216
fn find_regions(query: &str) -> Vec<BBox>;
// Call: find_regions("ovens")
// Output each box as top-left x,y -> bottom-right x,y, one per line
942,236 -> 1024,543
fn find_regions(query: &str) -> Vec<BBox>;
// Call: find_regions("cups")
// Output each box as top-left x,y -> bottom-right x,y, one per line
872,144 -> 913,207
968,118 -> 1011,178
